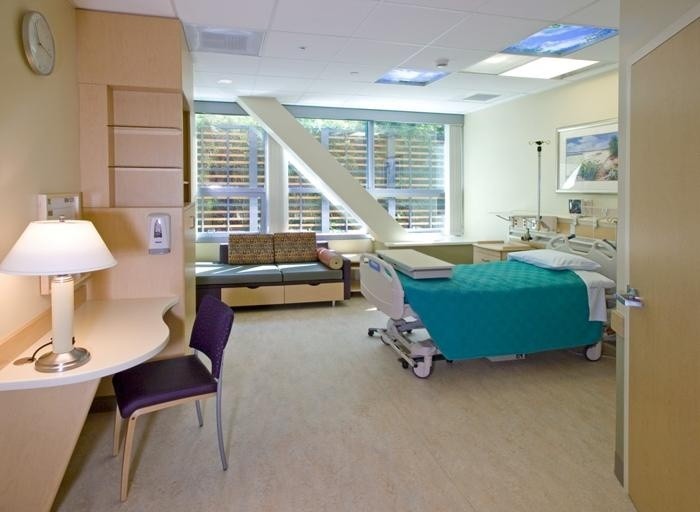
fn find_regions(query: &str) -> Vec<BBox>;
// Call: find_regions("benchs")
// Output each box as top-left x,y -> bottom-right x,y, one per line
195,241 -> 352,308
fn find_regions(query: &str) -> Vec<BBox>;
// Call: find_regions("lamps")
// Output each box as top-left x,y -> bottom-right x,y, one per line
0,216 -> 118,374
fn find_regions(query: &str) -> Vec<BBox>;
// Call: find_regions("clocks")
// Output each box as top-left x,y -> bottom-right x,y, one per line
22,10 -> 56,76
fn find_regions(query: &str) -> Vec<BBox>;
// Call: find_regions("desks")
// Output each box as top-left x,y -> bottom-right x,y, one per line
0,297 -> 179,391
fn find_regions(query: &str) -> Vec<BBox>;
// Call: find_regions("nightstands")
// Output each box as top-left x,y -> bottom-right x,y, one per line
473,240 -> 531,265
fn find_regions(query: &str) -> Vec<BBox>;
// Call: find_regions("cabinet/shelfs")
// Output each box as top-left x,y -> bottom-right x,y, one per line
108,125 -> 183,172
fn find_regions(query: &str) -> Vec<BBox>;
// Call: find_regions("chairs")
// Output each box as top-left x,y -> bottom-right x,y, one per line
111,295 -> 234,502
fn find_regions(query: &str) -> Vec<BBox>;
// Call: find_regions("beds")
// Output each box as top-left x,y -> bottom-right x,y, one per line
359,235 -> 617,379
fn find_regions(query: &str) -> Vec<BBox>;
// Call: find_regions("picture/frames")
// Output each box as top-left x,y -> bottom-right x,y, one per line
555,117 -> 619,194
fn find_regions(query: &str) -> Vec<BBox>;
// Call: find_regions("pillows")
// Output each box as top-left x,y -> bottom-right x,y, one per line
228,234 -> 275,264
274,232 -> 317,263
507,249 -> 601,272
316,247 -> 343,269
